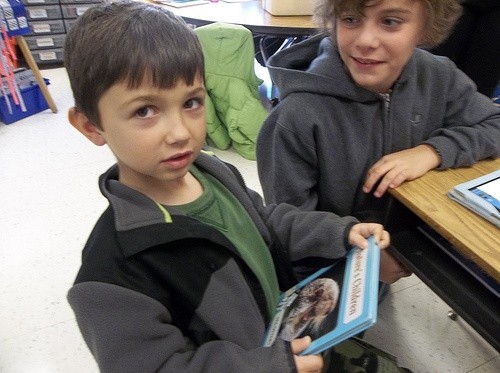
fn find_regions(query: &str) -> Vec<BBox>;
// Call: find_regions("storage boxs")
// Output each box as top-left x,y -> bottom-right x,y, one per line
1,75 -> 50,125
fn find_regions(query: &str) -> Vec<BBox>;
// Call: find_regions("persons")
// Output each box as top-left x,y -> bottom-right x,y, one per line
64,0 -> 390,373
256,0 -> 500,282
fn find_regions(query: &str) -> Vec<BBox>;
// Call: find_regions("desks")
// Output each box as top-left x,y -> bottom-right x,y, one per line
140,0 -> 334,67
350,152 -> 500,356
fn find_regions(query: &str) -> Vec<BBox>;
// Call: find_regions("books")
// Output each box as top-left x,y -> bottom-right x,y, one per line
447,169 -> 500,229
259,234 -> 381,356
